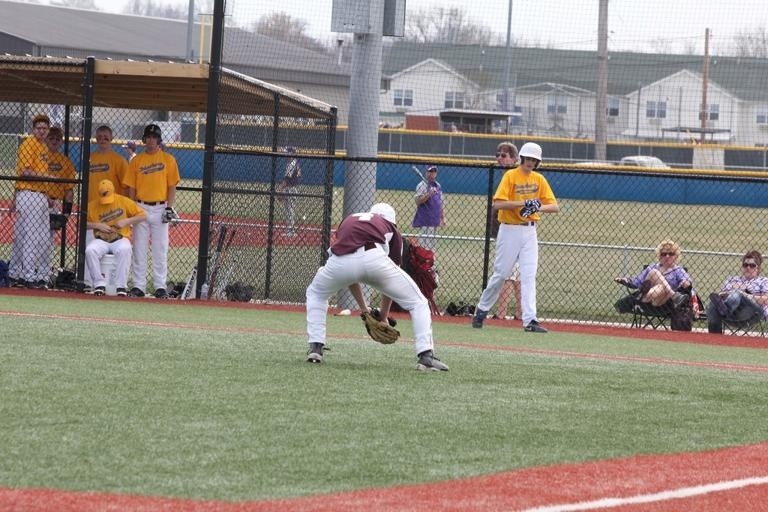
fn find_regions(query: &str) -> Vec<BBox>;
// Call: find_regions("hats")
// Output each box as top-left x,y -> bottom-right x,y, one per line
145,125 -> 161,136
32,115 -> 50,122
284,145 -> 296,154
423,164 -> 439,173
96,179 -> 115,205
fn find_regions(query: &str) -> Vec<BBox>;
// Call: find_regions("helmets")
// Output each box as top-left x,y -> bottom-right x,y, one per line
517,142 -> 543,170
369,203 -> 396,225
121,141 -> 136,150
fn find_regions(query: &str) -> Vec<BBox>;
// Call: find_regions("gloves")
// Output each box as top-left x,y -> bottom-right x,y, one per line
161,207 -> 173,223
520,207 -> 534,217
525,198 -> 541,210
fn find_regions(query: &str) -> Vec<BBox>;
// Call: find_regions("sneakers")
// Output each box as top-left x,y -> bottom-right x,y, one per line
306,343 -> 325,364
26,282 -> 38,288
153,288 -> 169,299
672,291 -> 690,310
93,286 -> 106,296
709,292 -> 728,315
6,278 -> 15,288
16,278 -> 25,288
416,352 -> 450,372
471,306 -> 489,328
38,279 -> 49,289
127,287 -> 145,297
524,320 -> 549,332
116,288 -> 127,297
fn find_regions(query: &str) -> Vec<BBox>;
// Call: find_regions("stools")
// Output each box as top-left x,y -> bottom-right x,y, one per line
98,252 -> 115,297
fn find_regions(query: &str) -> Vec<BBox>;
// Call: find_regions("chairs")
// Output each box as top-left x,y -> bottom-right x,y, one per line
615,265 -> 692,329
709,276 -> 767,336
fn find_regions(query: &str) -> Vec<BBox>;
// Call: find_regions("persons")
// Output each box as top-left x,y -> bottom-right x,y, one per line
304,202 -> 450,372
122,124 -> 181,298
470,141 -> 560,333
613,239 -> 692,331
158,141 -> 167,154
411,163 -> 448,248
705,250 -> 768,335
44,127 -> 75,286
123,138 -> 137,165
76,179 -> 147,297
15,114 -> 58,289
278,144 -> 301,237
86,126 -> 129,201
488,142 -> 522,321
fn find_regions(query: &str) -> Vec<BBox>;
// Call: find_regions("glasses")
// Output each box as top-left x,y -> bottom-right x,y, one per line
49,137 -> 60,142
495,152 -> 507,158
742,262 -> 757,268
524,157 -> 537,162
660,252 -> 675,256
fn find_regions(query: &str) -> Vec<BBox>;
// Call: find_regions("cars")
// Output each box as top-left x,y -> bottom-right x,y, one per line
619,153 -> 671,169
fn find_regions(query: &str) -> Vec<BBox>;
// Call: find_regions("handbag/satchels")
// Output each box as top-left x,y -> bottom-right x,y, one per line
688,287 -> 700,320
725,290 -> 742,312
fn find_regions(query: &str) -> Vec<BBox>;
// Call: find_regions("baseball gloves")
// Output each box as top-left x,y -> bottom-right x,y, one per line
50,213 -> 68,230
275,186 -> 283,202
95,230 -> 120,243
360,306 -> 400,343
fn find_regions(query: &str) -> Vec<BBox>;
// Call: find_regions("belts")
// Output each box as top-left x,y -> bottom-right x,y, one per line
137,199 -> 165,206
502,221 -> 535,227
16,188 -> 48,194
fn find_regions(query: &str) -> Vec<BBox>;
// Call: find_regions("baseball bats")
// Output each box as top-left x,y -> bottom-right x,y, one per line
412,164 -> 439,194
181,224 -> 251,301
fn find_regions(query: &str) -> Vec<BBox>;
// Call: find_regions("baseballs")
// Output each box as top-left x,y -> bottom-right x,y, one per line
621,221 -> 625,225
302,215 -> 306,220
334,191 -> 338,195
